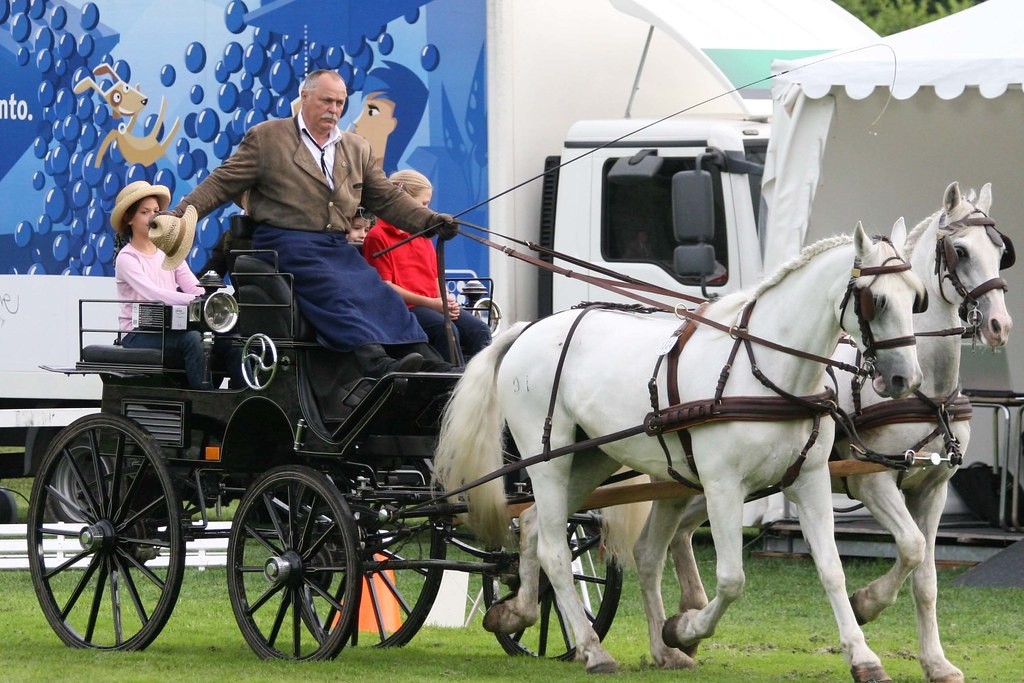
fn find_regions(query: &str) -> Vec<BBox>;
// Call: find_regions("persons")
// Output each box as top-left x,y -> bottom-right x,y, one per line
148,70 -> 492,404
110,181 -> 212,390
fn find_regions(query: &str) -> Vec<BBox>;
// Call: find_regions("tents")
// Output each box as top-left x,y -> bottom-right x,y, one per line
760,0 -> 1024,564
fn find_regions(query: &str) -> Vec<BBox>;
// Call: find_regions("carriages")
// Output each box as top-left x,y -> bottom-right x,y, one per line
25,181 -> 1016,682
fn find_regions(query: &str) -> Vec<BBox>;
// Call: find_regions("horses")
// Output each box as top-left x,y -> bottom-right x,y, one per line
429,217 -> 924,683
596,181 -> 1011,683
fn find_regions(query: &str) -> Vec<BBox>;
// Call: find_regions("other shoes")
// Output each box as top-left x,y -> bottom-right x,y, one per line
385,353 -> 424,376
441,365 -> 466,394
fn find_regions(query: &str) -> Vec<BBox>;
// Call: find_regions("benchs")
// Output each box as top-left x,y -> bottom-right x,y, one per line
228,212 -> 495,344
77,298 -> 214,390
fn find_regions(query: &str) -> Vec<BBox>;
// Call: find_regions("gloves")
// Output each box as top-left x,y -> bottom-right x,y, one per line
427,212 -> 459,240
147,209 -> 182,232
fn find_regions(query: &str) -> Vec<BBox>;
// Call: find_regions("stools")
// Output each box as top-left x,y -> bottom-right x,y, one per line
969,396 -> 1024,533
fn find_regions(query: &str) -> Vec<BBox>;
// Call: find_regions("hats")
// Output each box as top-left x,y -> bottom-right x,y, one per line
147,204 -> 200,271
109,180 -> 171,235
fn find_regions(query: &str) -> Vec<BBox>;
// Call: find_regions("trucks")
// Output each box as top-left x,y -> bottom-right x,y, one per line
0,0 -> 770,537
